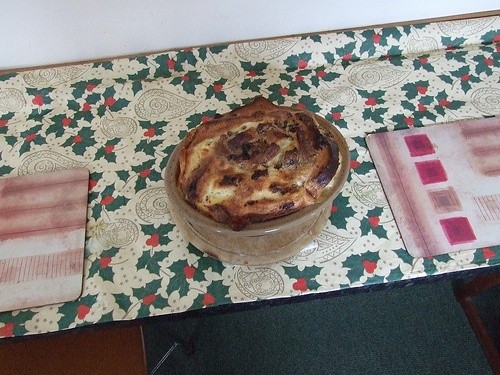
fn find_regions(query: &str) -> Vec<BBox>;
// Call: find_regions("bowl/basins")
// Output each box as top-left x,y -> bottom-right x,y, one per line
163,107 -> 350,265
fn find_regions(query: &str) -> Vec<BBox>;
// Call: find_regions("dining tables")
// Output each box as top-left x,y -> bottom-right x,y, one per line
0,10 -> 500,375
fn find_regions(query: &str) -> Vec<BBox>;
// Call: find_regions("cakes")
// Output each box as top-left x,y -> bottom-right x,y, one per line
175,96 -> 339,232
